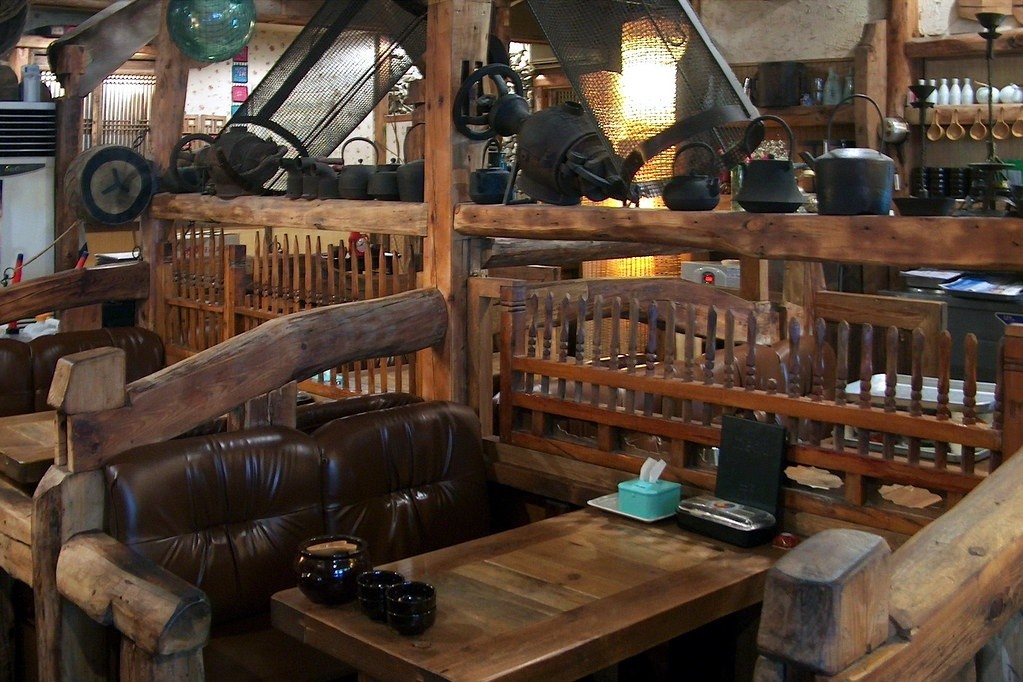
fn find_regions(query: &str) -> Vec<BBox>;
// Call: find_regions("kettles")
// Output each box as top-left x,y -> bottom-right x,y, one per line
338,137 -> 379,200
735,115 -> 810,213
799,94 -> 895,215
663,143 -> 721,211
469,139 -> 515,204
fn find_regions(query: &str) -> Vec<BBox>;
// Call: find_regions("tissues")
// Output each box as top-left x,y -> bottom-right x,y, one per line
618,456 -> 682,520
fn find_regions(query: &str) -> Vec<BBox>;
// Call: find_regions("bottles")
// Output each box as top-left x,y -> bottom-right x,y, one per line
366,154 -> 425,202
295,536 -> 372,604
803,169 -> 815,193
950,77 -> 961,105
906,78 -> 938,107
961,78 -> 974,104
802,67 -> 854,105
938,78 -> 949,105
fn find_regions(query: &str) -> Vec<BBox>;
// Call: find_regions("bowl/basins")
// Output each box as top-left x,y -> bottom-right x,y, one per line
892,195 -> 966,216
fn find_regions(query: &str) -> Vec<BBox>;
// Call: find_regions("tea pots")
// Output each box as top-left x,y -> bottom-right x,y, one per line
973,80 -> 999,103
999,82 -> 1023,103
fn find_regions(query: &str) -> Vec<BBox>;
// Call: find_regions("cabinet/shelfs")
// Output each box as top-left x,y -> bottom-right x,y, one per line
887,0 -> 1023,209
675,20 -> 887,207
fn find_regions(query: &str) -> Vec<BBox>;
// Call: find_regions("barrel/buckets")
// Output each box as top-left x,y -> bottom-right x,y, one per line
911,166 -> 971,197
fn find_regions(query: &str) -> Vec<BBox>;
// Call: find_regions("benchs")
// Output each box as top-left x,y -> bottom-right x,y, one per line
0,211 -> 1023,682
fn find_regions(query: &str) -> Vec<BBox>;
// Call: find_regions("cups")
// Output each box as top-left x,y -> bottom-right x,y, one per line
355,570 -> 405,621
385,580 -> 437,632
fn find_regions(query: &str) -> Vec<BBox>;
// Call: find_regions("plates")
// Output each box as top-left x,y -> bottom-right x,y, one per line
587,493 -> 676,524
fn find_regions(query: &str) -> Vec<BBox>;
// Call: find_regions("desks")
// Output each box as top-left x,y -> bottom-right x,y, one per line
0,388 -> 314,496
269,506 -> 802,681
